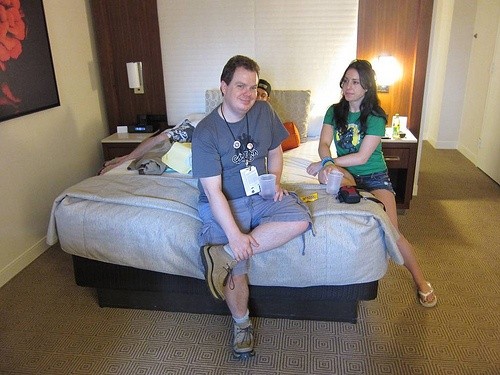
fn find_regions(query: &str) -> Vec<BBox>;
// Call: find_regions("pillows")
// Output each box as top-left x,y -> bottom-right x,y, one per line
205,89 -> 312,142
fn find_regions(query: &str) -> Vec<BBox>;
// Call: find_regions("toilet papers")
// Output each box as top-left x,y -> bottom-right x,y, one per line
392,116 -> 407,135
126,62 -> 144,89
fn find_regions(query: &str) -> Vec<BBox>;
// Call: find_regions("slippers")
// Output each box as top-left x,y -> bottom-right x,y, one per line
418,280 -> 437,307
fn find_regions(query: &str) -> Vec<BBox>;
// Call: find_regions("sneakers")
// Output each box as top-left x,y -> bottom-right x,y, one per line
233,319 -> 256,358
201,244 -> 238,303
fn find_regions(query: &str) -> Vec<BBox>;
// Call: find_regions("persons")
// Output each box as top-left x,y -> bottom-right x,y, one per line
98,79 -> 271,176
307,60 -> 438,308
192,55 -> 317,361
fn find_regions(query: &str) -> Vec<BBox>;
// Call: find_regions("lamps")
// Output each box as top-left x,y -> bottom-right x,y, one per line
371,55 -> 403,93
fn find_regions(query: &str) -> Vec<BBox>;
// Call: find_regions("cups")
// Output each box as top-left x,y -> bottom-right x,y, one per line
326,170 -> 344,195
258,174 -> 276,200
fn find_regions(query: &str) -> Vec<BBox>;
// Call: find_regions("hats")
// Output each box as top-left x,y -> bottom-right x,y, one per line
257,79 -> 271,96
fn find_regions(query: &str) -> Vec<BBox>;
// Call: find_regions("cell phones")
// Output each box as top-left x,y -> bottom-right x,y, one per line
240,165 -> 261,196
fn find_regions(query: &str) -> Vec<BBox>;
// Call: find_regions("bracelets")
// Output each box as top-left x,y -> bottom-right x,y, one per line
321,156 -> 334,167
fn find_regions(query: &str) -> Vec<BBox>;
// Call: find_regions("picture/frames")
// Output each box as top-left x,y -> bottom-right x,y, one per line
0,0 -> 61,122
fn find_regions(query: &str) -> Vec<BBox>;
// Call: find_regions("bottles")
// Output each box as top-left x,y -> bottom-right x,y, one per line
392,114 -> 400,141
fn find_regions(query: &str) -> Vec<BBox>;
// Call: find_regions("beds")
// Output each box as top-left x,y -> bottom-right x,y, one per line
47,117 -> 403,324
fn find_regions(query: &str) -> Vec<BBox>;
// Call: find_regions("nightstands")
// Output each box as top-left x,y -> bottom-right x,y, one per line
381,126 -> 418,215
101,129 -> 160,165
